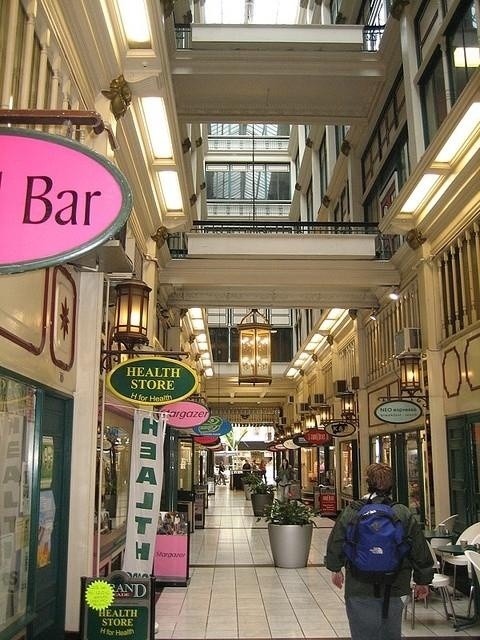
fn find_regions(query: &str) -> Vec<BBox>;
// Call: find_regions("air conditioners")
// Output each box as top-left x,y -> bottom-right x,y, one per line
392,325 -> 420,356
333,379 -> 346,394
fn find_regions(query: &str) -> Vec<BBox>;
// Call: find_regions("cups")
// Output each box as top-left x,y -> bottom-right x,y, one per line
439,524 -> 445,534
460,540 -> 468,550
474,543 -> 480,549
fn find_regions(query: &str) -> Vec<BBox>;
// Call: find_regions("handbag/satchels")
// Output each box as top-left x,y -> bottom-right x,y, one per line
275,476 -> 281,483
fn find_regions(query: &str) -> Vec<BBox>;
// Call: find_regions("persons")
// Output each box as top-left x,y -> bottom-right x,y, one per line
277,458 -> 296,502
242,459 -> 251,474
324,462 -> 435,639
259,460 -> 267,485
216,461 -> 227,485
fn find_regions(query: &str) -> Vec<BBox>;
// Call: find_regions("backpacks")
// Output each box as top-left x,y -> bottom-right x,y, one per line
343,499 -> 410,586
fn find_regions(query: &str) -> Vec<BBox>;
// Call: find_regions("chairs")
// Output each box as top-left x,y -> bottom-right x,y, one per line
405,512 -> 480,631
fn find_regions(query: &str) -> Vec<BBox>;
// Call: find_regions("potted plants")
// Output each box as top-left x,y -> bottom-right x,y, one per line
241,473 -> 322,569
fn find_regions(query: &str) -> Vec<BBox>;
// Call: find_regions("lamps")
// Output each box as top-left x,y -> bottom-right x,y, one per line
236,307 -> 274,386
391,347 -> 427,395
270,391 -> 357,441
103,263 -> 158,353
